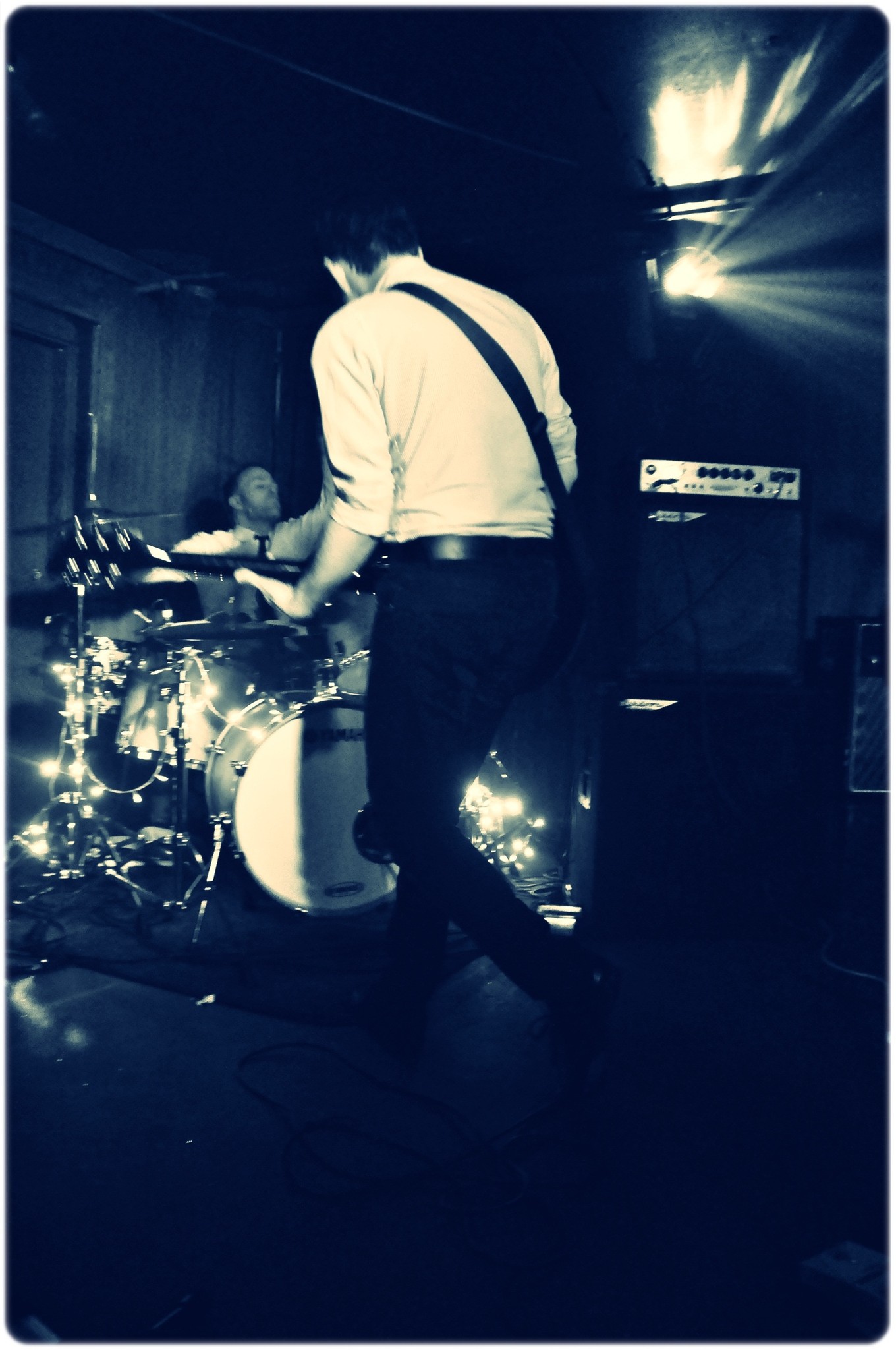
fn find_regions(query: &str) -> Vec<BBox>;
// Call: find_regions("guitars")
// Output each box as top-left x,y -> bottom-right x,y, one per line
44,516 -> 384,596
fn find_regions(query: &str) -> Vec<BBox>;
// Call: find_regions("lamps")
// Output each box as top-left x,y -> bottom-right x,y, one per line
651,218 -> 726,299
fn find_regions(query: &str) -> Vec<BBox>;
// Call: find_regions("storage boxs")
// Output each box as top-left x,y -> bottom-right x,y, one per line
565,670 -> 820,927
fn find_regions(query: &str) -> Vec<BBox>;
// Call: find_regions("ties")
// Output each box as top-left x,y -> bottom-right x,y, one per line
253,534 -> 271,561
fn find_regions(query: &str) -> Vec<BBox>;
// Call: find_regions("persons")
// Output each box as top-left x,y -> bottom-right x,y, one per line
229,188 -> 612,1050
157,461 -> 330,584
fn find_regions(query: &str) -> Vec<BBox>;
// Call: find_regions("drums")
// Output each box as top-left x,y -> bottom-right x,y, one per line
68,635 -> 136,715
116,642 -> 273,774
334,649 -> 369,694
205,687 -> 400,915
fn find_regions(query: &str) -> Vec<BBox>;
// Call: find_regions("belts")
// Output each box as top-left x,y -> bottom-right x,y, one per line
398,534 -> 559,563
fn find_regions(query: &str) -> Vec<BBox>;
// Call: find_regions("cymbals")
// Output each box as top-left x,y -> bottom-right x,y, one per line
12,512 -> 159,534
143,614 -> 299,638
